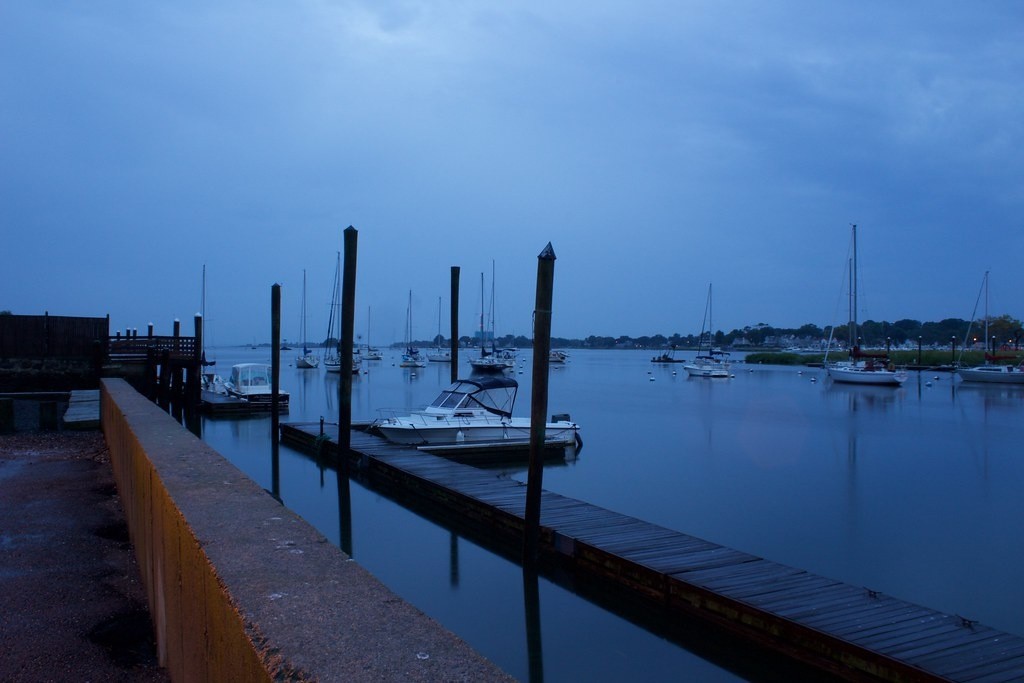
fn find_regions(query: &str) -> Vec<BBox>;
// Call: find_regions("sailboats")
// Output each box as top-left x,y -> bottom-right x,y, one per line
322,252 -> 383,375
820,224 -> 909,386
462,261 -> 522,374
682,283 -> 732,379
294,269 -> 321,369
156,263 -> 249,418
399,290 -> 430,369
424,296 -> 452,362
951,270 -> 1023,385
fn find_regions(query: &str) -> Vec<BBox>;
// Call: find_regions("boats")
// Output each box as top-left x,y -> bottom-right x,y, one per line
650,350 -> 687,364
223,362 -> 291,412
374,370 -> 584,462
548,348 -> 571,364
